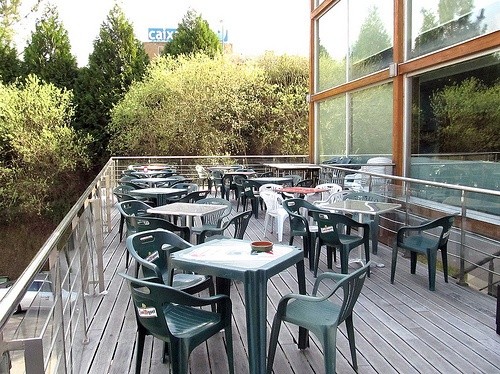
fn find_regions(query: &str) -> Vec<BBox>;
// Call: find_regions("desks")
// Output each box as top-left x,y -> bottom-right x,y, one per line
262,162 -> 321,185
246,176 -> 293,210
320,200 -> 402,254
131,178 -> 179,188
146,202 -> 229,244
272,187 -> 331,205
134,171 -> 168,178
160,237 -> 310,374
130,188 -> 188,207
224,171 -> 270,179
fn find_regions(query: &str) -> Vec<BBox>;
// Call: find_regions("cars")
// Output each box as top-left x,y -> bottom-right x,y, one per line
320,155 -> 500,203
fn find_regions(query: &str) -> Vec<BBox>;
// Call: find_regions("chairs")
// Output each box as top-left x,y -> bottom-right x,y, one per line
113,157 -> 460,374
